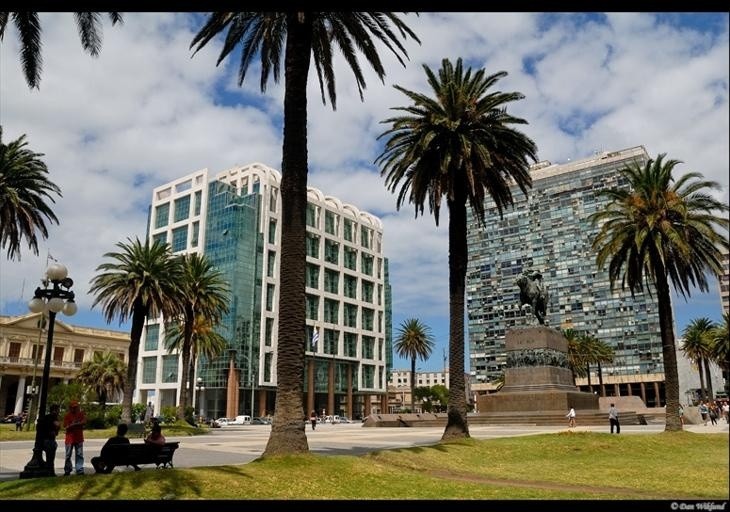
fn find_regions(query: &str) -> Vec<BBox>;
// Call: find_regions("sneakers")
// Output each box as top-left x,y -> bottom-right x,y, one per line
63,472 -> 69,475
77,472 -> 85,475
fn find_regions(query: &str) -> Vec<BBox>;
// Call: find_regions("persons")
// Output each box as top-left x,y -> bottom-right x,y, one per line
92,424 -> 130,475
14,414 -> 22,432
565,407 -> 576,429
522,258 -> 547,300
607,404 -> 621,434
144,402 -> 154,423
135,412 -> 144,425
36,405 -> 62,476
62,399 -> 88,476
310,409 -> 317,431
677,400 -> 730,430
131,423 -> 166,472
20,411 -> 29,428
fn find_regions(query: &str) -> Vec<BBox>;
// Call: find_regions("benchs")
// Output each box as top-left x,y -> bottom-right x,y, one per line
93,441 -> 181,473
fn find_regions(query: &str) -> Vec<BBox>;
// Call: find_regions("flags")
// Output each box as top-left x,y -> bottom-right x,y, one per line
311,322 -> 320,348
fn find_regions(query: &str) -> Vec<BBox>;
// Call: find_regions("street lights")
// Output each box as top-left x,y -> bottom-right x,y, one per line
194,377 -> 205,424
17,264 -> 78,480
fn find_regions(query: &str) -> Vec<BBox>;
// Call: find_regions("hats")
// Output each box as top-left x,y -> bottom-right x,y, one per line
69,400 -> 80,407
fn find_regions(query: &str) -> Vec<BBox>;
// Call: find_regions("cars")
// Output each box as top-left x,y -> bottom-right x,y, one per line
305,415 -> 353,424
2,415 -> 19,422
215,415 -> 272,424
